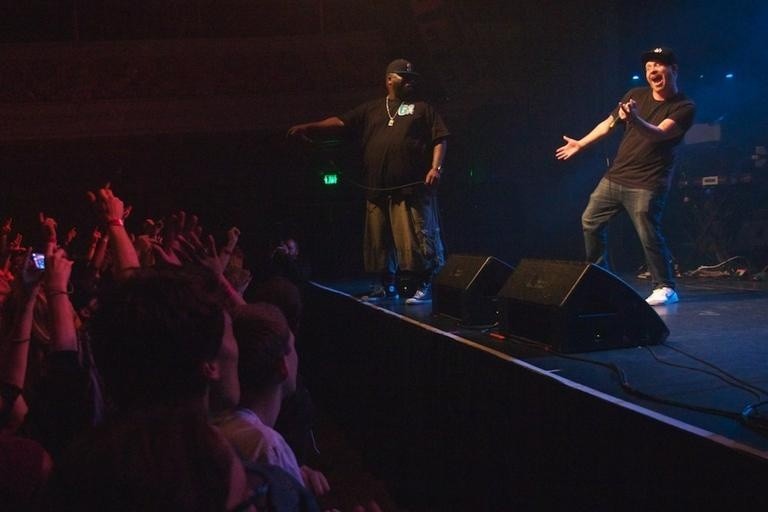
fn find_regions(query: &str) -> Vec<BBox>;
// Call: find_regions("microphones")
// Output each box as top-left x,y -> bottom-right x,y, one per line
610,116 -> 620,129
299,134 -> 313,145
745,155 -> 761,161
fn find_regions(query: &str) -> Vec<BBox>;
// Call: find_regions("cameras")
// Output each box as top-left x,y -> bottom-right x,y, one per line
31,250 -> 47,272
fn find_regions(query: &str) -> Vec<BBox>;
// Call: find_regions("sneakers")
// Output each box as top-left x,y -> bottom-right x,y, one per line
405,288 -> 432,304
361,287 -> 386,301
645,287 -> 679,306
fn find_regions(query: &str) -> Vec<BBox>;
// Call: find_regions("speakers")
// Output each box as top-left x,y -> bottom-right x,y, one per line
432,255 -> 516,322
500,258 -> 670,353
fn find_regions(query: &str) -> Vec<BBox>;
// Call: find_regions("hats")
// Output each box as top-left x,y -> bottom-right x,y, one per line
640,46 -> 680,65
386,58 -> 421,76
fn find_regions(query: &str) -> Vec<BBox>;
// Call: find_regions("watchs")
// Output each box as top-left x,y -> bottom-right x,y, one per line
431,165 -> 445,174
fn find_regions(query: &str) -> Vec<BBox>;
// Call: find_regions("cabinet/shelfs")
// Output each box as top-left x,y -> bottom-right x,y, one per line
679,188 -> 735,273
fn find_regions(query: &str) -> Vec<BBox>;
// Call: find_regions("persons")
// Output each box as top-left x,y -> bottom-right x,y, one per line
555,47 -> 696,306
1,182 -> 331,512
286,59 -> 448,305
